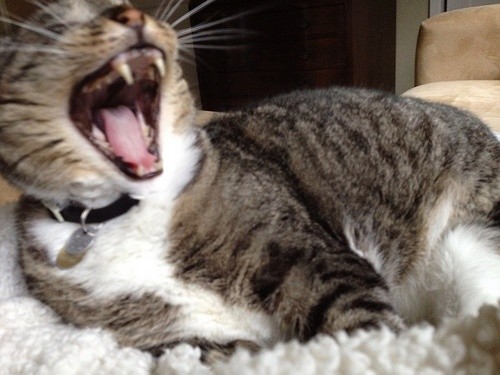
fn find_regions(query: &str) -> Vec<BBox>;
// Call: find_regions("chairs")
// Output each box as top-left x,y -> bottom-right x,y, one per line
414,4 -> 500,86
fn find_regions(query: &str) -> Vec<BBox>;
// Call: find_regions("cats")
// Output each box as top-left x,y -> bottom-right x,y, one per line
0,0 -> 500,366
